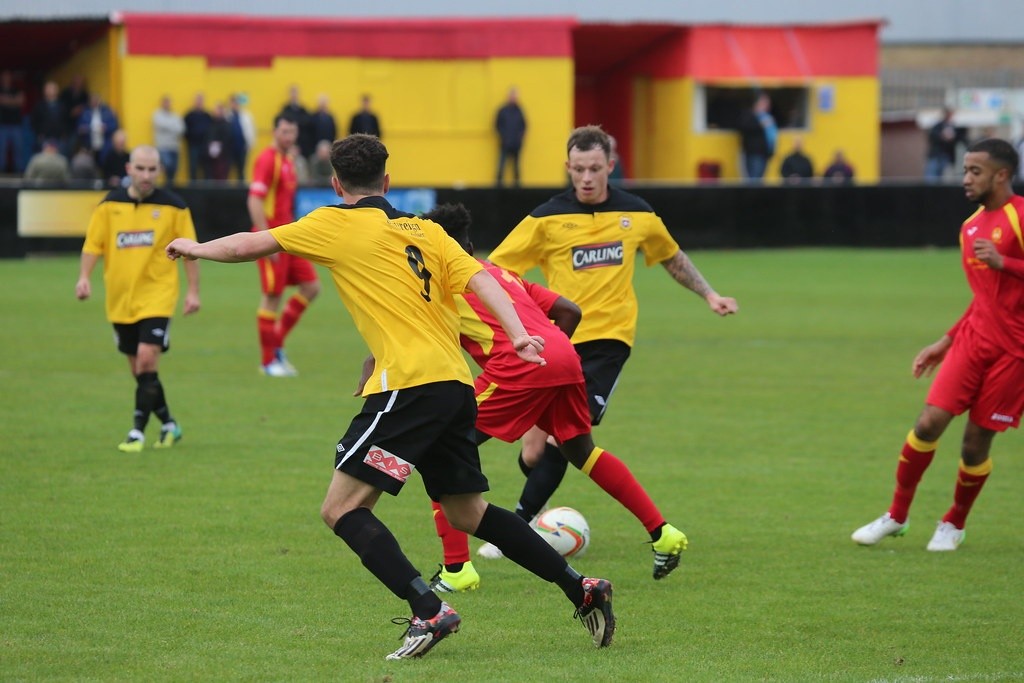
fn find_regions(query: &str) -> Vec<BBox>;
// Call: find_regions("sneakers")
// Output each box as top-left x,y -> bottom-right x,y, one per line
152,418 -> 183,448
258,348 -> 299,378
118,432 -> 144,454
925,521 -> 967,551
476,541 -> 503,560
384,602 -> 462,663
644,523 -> 687,580
852,513 -> 909,545
574,576 -> 614,650
429,559 -> 479,593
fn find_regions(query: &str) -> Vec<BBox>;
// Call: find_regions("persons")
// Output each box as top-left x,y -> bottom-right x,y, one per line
352,204 -> 686,592
349,99 -> 380,138
0,72 -> 131,190
852,138 -> 1024,550
496,90 -> 526,186
822,154 -> 853,185
166,134 -> 616,658
780,144 -> 814,186
248,117 -> 320,375
926,111 -> 958,179
284,87 -> 337,186
154,95 -> 256,182
476,127 -> 737,558
739,95 -> 776,178
76,148 -> 202,454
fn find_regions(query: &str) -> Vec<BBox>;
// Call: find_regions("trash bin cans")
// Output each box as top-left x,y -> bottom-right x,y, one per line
697,161 -> 720,182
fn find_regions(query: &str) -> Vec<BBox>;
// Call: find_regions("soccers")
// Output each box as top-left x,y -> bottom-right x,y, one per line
532,506 -> 590,560
